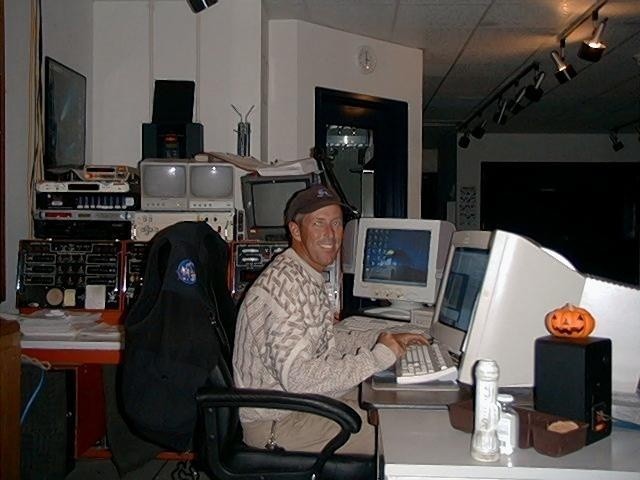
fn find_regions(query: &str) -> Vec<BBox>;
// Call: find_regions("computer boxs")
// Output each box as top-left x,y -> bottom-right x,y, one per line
20,364 -> 76,479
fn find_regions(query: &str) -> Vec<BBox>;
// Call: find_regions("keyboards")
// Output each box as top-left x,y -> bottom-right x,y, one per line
395,342 -> 458,386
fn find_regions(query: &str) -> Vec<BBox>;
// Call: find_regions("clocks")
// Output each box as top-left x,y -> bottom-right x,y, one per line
355,44 -> 378,75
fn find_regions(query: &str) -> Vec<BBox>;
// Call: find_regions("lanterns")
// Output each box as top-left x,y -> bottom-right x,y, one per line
545,304 -> 595,338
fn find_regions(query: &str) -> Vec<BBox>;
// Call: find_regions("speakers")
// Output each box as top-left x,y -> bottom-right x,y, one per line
533,338 -> 613,446
140,125 -> 205,159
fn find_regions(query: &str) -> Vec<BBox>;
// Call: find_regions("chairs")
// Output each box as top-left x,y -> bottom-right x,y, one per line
128,219 -> 383,478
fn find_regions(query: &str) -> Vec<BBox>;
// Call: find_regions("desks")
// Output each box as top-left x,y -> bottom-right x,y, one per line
359,376 -> 640,475
13,305 -> 201,462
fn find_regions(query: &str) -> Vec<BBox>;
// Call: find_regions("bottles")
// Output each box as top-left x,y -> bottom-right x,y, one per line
496,393 -> 519,468
470,359 -> 500,464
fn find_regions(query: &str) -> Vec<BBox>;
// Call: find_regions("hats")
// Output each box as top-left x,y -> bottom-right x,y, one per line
285,185 -> 352,221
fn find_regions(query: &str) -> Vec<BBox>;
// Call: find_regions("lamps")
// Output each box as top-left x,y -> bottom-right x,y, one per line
493,101 -> 508,127
508,89 -> 526,115
549,38 -> 577,84
577,10 -> 607,62
525,71 -> 545,102
470,120 -> 488,141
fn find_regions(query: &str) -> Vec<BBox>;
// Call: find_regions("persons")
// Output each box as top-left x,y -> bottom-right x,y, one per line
232,186 -> 430,455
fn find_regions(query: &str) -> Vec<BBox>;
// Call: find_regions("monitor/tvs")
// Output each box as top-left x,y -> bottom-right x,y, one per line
240,173 -> 320,242
188,163 -> 236,212
140,161 -> 188,210
41,55 -> 88,170
352,218 -> 456,322
430,230 -> 640,392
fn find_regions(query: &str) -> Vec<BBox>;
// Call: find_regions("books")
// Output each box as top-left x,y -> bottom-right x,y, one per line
206,151 -> 319,177
0,308 -> 121,336
